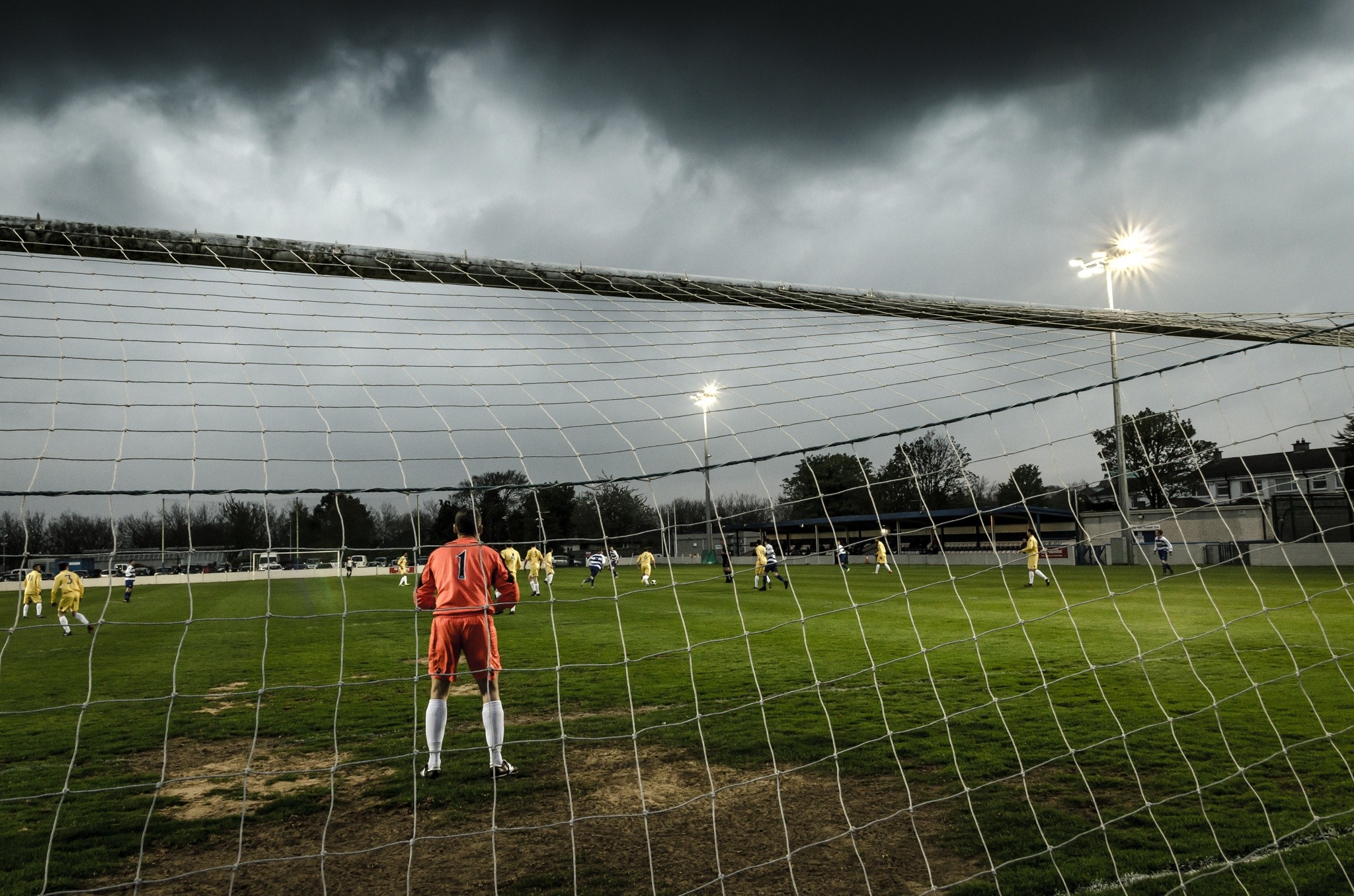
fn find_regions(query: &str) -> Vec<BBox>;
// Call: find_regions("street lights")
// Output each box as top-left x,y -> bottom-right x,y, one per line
1064,228 -> 1161,567
691,379 -> 727,564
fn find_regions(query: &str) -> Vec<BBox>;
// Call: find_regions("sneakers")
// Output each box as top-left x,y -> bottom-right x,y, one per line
489,759 -> 520,779
419,764 -> 441,778
21,599 -> 135,635
398,567 -> 893,614
1025,569 -> 1174,588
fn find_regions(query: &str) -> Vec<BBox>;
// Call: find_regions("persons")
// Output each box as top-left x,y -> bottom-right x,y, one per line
123,559 -> 139,603
412,508 -> 521,779
51,561 -> 94,636
344,528 -> 1175,617
22,563 -> 47,618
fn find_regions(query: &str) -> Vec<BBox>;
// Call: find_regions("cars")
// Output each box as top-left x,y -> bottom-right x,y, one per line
0,547 -> 584,583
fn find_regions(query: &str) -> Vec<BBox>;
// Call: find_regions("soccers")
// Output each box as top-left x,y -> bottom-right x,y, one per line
650,580 -> 657,585
